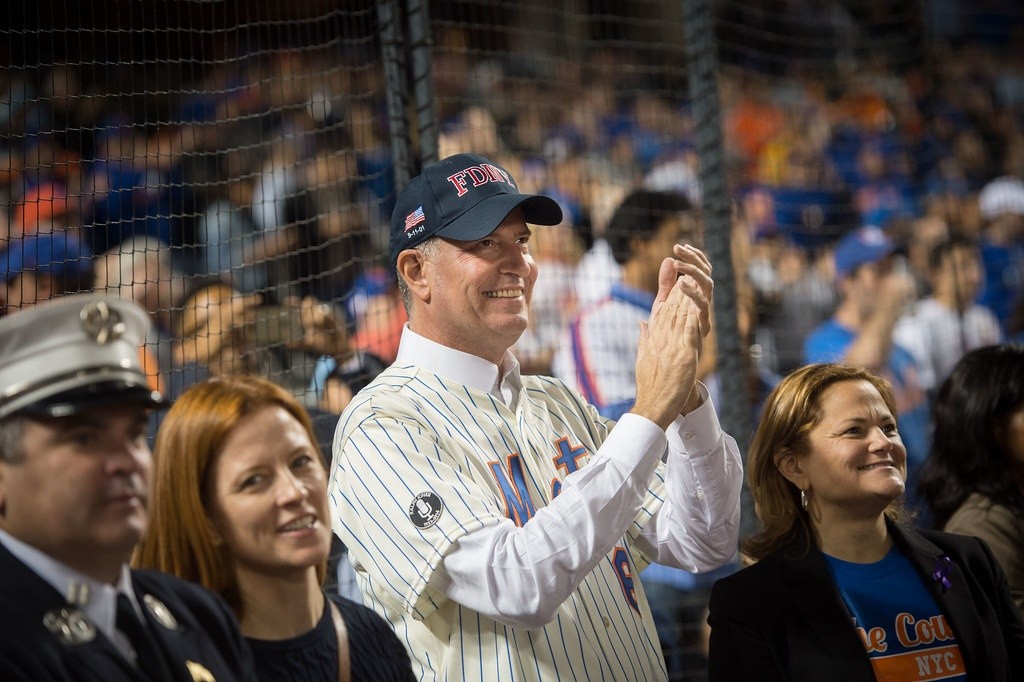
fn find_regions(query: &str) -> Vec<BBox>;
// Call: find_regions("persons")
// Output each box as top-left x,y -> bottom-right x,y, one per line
907,344 -> 1024,637
130,373 -> 419,682
0,294 -> 254,682
0,0 -> 407,473
707,363 -> 1024,682
408,0 -> 1024,595
326,152 -> 744,682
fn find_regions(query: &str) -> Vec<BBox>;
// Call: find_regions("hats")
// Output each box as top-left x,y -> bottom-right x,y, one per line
0,293 -> 174,422
834,224 -> 914,281
0,233 -> 96,290
977,175 -> 1024,222
387,153 -> 564,263
